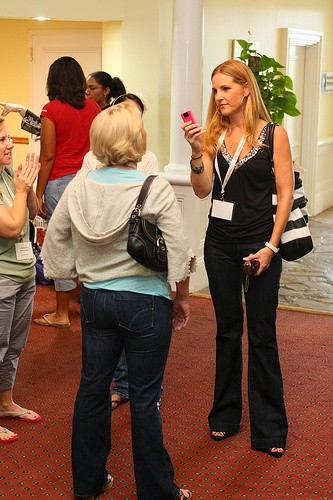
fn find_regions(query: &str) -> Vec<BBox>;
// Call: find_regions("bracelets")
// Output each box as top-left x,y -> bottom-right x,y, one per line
189,159 -> 204,174
19,105 -> 22,112
191,154 -> 203,160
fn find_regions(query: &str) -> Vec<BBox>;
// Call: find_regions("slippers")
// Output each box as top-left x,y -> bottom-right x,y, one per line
0,409 -> 41,423
111,393 -> 123,409
32,314 -> 71,328
0,427 -> 19,444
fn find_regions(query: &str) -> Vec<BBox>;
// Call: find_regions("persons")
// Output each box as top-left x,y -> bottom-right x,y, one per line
181,60 -> 295,458
40,100 -> 199,500
0,56 -> 163,409
0,117 -> 42,442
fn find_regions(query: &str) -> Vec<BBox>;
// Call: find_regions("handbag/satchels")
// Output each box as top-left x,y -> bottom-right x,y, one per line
127,175 -> 169,273
265,121 -> 315,262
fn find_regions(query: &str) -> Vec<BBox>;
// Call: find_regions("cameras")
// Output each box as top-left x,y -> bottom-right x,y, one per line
243,251 -> 258,276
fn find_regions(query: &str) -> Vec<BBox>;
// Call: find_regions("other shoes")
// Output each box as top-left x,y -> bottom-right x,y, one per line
81,472 -> 113,500
178,487 -> 192,500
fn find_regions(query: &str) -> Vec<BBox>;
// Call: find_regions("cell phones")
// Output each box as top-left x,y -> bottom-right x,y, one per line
181,110 -> 200,135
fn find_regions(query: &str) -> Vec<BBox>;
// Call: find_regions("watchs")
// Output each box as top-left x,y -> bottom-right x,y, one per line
265,242 -> 280,254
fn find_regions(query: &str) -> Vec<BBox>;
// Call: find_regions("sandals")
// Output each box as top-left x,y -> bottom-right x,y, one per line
211,429 -> 235,441
264,444 -> 285,458
